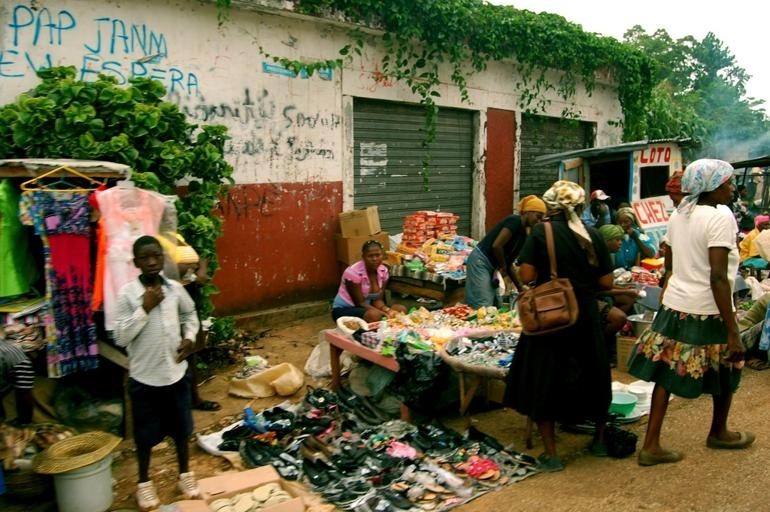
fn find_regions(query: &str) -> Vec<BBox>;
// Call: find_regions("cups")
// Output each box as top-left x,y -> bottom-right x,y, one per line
629,386 -> 647,401
390,265 -> 441,284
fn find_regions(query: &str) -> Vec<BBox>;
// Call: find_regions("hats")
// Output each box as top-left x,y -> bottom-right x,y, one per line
31,431 -> 123,474
591,190 -> 611,201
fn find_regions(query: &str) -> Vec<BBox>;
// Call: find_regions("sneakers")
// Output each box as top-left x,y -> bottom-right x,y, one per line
174,471 -> 200,500
537,452 -> 564,471
587,438 -> 608,457
134,477 -> 161,510
218,386 -> 502,512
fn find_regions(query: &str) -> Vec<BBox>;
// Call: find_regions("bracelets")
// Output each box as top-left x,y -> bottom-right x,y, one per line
380,304 -> 387,310
385,307 -> 391,313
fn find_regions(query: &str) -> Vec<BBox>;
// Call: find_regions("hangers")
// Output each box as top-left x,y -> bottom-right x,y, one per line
20,165 -> 136,192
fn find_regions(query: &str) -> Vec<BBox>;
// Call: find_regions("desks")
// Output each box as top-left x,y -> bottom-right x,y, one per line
323,266 -> 533,450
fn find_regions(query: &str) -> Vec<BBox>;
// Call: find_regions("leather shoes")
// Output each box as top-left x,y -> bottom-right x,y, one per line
706,430 -> 757,448
637,447 -> 685,468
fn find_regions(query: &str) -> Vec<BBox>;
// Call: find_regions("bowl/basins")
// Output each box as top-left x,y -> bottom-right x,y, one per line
626,313 -> 659,337
608,391 -> 638,416
5,472 -> 53,508
734,275 -> 750,297
636,285 -> 664,311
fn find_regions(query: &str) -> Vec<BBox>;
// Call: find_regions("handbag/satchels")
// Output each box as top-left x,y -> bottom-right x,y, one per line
517,278 -> 579,336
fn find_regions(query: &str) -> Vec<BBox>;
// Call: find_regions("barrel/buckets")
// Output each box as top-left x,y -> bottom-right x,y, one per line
53,453 -> 115,512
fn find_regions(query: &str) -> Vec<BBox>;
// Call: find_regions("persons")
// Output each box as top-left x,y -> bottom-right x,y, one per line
502,179 -> 613,472
113,235 -> 200,507
580,167 -> 770,370
0,340 -> 37,498
331,240 -> 407,324
465,195 -> 547,311
626,158 -> 757,470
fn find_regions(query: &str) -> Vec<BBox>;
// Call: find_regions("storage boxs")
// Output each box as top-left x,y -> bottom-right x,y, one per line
337,205 -> 382,239
337,230 -> 390,266
615,337 -> 638,372
177,463 -> 305,512
